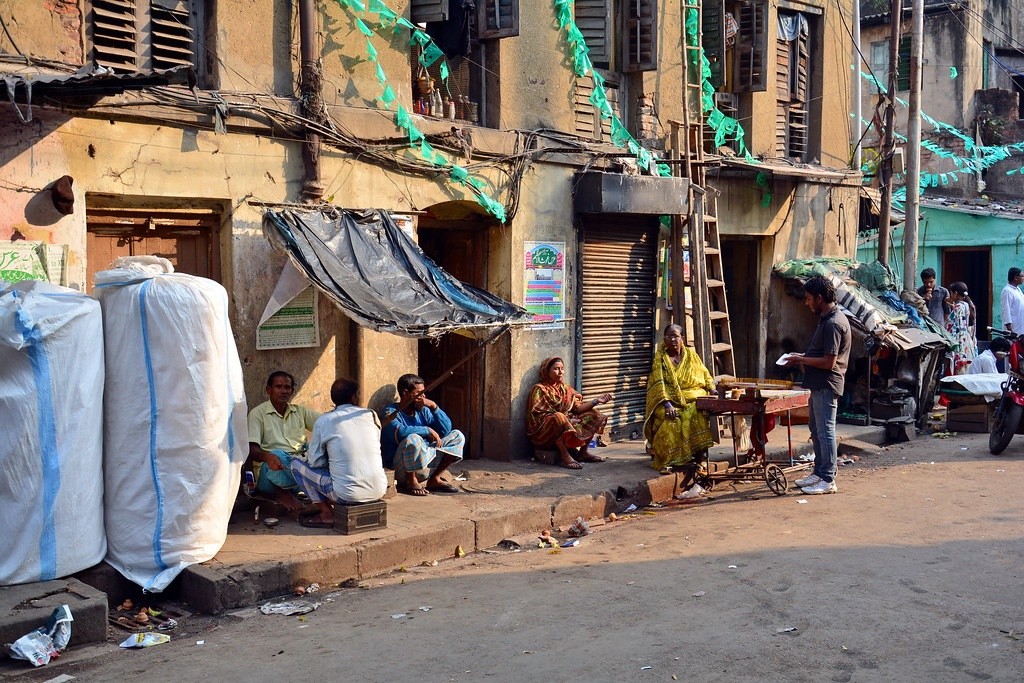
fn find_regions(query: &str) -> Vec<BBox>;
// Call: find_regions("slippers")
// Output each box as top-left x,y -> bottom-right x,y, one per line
299,511 -> 335,529
562,461 -> 583,469
578,456 -> 604,463
407,485 -> 427,496
660,467 -> 673,473
425,482 -> 459,493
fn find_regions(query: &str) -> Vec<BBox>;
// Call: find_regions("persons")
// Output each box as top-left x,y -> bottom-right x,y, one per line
783,274 -> 851,495
642,324 -> 718,474
526,356 -> 612,469
247,371 -> 388,530
1002,267 -> 1024,344
916,266 -> 979,407
967,336 -> 1011,375
380,373 -> 465,496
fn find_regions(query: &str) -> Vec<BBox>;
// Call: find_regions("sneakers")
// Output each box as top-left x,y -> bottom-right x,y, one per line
801,479 -> 837,495
794,475 -> 823,486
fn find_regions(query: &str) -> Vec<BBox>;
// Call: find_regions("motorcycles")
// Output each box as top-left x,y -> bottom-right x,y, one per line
985,325 -> 1024,455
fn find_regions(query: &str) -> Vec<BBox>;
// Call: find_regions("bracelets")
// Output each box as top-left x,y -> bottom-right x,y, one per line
665,403 -> 671,408
592,399 -> 597,406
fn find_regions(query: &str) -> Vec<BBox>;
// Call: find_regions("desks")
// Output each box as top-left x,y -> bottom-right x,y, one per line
696,389 -> 811,489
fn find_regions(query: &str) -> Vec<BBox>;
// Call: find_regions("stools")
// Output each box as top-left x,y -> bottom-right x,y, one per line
383,468 -> 397,500
332,499 -> 387,535
533,447 -> 579,465
